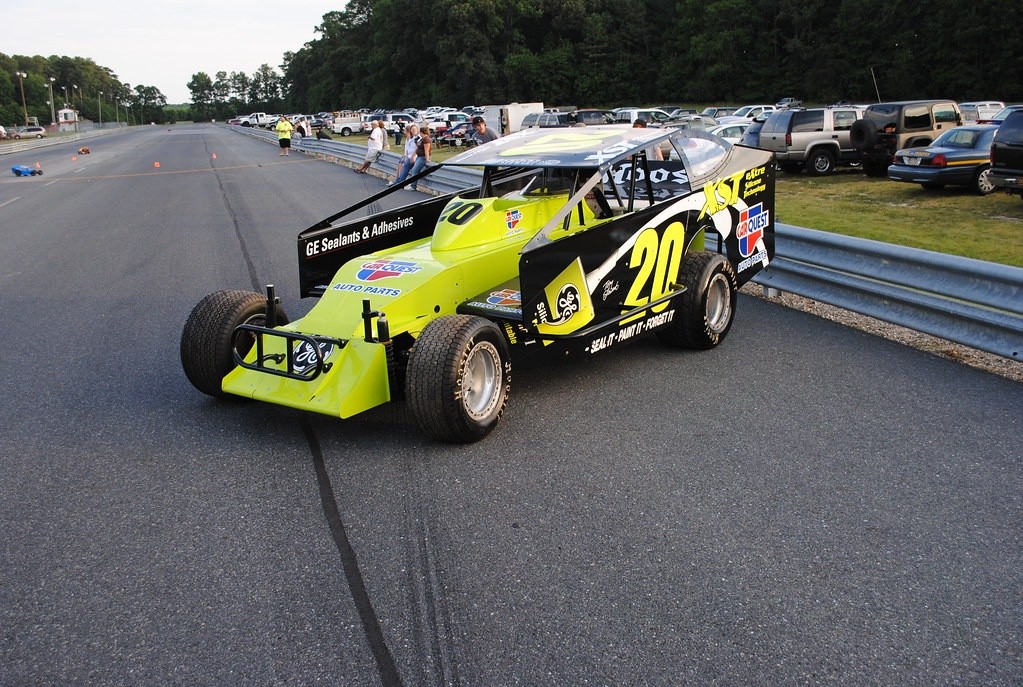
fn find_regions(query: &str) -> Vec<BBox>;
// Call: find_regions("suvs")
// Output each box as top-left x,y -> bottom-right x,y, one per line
987,110 -> 1023,202
850,99 -> 965,178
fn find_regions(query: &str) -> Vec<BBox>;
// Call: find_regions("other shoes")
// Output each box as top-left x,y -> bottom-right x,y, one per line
361,171 -> 366,174
354,169 -> 361,173
403,184 -> 415,190
388,181 -> 393,186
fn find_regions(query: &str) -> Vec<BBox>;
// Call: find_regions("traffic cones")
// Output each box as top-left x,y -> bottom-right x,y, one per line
212,153 -> 218,160
35,161 -> 41,169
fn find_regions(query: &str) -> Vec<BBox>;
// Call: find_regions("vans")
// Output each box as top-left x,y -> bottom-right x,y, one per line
956,100 -> 1005,129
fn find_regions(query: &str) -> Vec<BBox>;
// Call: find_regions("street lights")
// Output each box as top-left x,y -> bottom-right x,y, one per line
98,91 -> 104,129
16,72 -> 28,127
43,78 -> 56,123
116,96 -> 120,125
72,84 -> 78,112
61,85 -> 70,110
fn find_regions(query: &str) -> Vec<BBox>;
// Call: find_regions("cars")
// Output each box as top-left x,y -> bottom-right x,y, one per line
886,124 -> 1000,196
10,126 -> 48,140
990,104 -> 1023,128
228,104 -> 488,148
519,102 -> 873,177
775,97 -> 802,108
827,100 -> 850,110
178,122 -> 776,445
11,164 -> 43,177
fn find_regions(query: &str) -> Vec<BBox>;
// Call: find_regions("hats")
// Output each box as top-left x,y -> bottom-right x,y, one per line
472,116 -> 483,125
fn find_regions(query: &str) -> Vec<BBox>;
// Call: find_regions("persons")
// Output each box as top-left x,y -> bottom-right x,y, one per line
276,116 -> 294,156
389,124 -> 431,191
294,118 -> 313,137
354,120 -> 383,174
472,116 -> 501,146
378,120 -> 388,147
394,118 -> 405,147
316,127 -> 333,140
419,115 -> 429,127
627,120 -> 663,161
